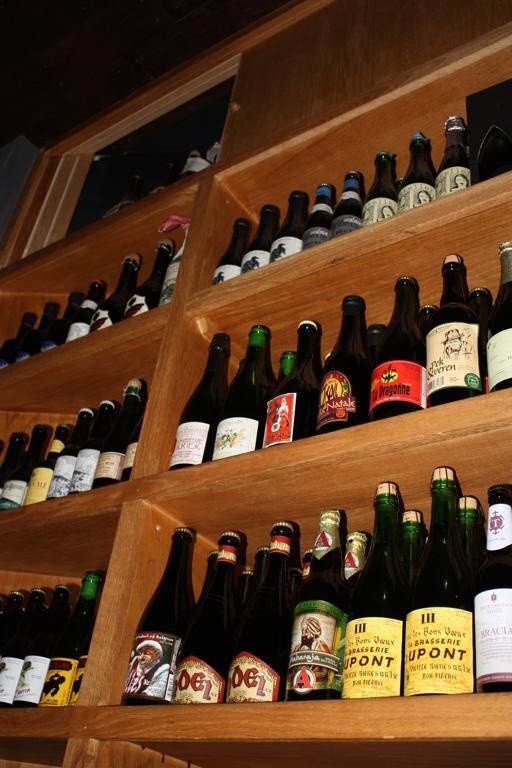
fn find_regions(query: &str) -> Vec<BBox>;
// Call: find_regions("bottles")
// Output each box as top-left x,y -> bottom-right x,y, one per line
209,115 -> 486,285
1,220 -> 189,369
167,240 -> 511,468
0,570 -> 101,712
122,464 -> 512,704
1,376 -> 144,510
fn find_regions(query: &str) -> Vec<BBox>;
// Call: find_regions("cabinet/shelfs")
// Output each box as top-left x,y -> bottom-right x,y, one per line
0,1 -> 512,767
